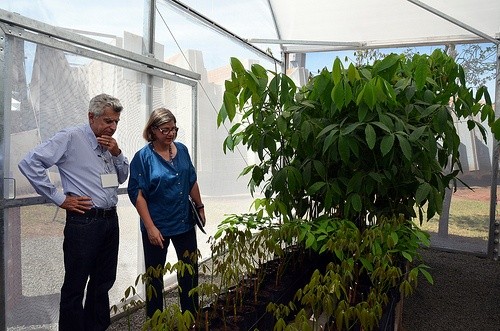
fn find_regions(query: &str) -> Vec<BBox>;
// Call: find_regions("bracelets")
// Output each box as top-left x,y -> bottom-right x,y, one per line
195,204 -> 204,209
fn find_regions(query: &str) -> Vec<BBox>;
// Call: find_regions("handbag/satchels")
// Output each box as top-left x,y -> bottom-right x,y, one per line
189,195 -> 207,235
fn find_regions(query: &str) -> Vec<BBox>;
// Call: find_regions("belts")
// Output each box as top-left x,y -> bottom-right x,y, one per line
69,206 -> 116,218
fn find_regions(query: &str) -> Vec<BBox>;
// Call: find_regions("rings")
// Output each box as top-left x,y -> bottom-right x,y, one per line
106,142 -> 109,146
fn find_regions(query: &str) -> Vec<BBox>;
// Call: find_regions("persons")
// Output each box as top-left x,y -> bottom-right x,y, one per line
18,93 -> 129,331
127,109 -> 206,321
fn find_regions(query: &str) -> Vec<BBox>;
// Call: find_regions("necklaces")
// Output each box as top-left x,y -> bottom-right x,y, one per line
151,142 -> 173,161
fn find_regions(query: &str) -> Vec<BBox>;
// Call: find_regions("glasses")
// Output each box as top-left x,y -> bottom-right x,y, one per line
155,125 -> 179,135
